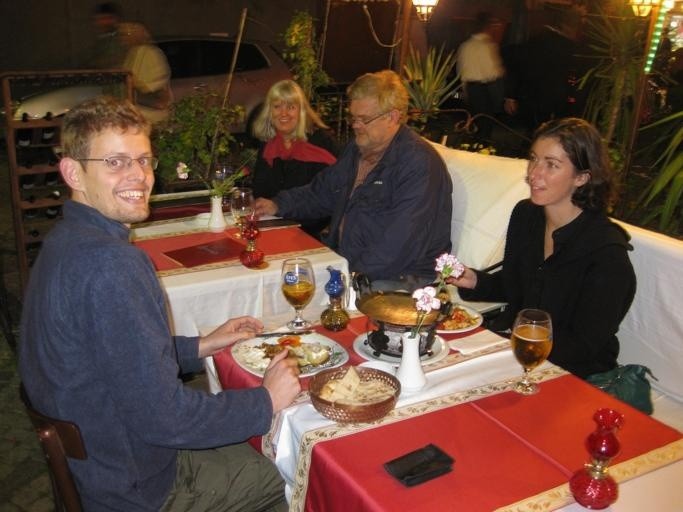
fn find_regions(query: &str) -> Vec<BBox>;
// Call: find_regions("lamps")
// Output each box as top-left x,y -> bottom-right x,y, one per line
412,0 -> 437,23
625,1 -> 654,21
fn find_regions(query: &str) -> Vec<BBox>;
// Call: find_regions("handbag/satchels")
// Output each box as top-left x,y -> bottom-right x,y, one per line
580,364 -> 652,415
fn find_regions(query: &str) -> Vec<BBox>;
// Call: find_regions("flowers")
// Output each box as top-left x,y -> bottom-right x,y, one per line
176,150 -> 259,198
412,252 -> 464,339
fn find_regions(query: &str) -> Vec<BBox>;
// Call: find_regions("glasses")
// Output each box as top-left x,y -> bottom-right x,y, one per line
75,156 -> 159,171
343,107 -> 400,128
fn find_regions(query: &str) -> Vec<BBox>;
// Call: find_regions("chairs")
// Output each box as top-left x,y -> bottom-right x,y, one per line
421,135 -> 531,314
19,381 -> 87,512
608,216 -> 683,433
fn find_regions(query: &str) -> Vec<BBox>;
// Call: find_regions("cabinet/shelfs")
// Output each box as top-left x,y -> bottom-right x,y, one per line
1,69 -> 133,308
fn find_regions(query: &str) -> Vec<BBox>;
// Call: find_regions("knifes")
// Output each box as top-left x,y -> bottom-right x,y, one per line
256,327 -> 313,338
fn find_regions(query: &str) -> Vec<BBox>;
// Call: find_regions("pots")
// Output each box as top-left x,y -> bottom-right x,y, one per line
352,274 -> 453,331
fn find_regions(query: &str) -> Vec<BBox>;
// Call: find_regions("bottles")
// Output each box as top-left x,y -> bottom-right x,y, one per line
21,162 -> 34,190
25,194 -> 36,219
44,192 -> 59,219
18,113 -> 32,148
40,112 -> 54,143
26,229 -> 40,267
43,162 -> 57,186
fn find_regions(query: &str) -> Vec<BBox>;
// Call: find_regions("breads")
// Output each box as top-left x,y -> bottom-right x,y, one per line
321,364 -> 394,404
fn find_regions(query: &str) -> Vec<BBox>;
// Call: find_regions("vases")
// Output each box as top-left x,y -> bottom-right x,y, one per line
208,196 -> 227,233
395,332 -> 428,392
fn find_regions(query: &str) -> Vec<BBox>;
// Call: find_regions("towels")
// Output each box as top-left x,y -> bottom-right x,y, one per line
383,443 -> 455,487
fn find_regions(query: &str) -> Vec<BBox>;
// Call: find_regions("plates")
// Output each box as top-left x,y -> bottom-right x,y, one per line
229,332 -> 349,380
433,304 -> 483,334
351,328 -> 449,366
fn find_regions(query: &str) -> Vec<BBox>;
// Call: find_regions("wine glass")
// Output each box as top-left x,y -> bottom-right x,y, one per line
279,258 -> 316,330
511,308 -> 552,395
229,187 -> 255,239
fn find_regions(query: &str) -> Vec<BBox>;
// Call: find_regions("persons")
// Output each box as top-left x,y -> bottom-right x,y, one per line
456,12 -> 507,128
18,94 -> 303,512
254,69 -> 454,294
250,78 -> 336,236
117,23 -> 173,109
445,117 -> 637,381
